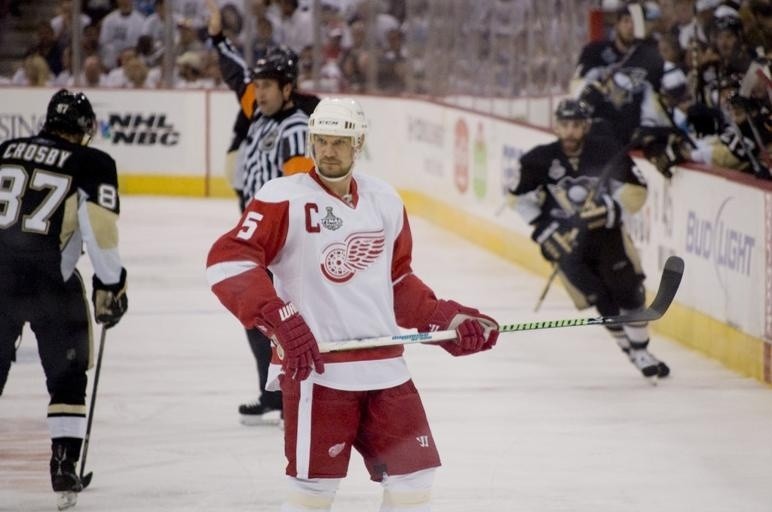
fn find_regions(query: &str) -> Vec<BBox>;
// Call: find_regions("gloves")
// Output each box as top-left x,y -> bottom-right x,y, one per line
418,300 -> 500,358
573,195 -> 618,229
253,302 -> 326,381
91,268 -> 129,329
533,224 -> 585,260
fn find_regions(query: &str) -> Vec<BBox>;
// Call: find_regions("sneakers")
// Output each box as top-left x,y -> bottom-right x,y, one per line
648,353 -> 669,377
629,349 -> 658,377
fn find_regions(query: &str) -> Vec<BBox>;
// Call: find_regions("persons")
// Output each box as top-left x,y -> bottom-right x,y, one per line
447,2 -> 620,95
0,0 -> 433,97
573,1 -> 772,179
1,85 -> 130,493
203,94 -> 501,510
502,96 -> 671,381
236,53 -> 314,419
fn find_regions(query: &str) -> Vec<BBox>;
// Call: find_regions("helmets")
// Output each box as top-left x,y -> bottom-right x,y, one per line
249,44 -> 300,92
551,99 -> 596,140
705,5 -> 746,55
304,96 -> 368,158
43,89 -> 98,148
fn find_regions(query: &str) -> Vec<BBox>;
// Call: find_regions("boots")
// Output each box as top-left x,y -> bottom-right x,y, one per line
239,391 -> 283,414
49,438 -> 85,493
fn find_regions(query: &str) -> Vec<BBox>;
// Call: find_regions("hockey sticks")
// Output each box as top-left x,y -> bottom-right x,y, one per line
80,322 -> 108,487
534,126 -> 671,314
277,256 -> 684,361
